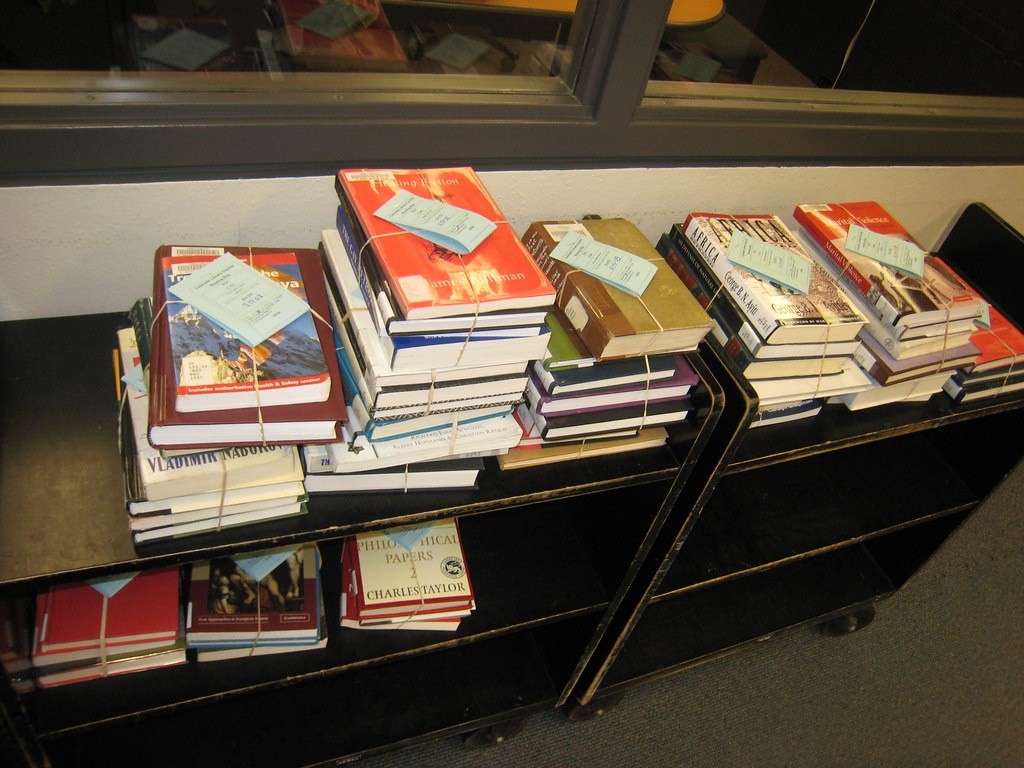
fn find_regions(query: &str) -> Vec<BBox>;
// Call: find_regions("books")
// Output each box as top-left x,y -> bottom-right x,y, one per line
127,0 -> 410,75
657,211 -> 873,430
335,164 -> 558,373
497,218 -> 714,473
302,227 -> 524,495
31,565 -> 187,690
922,255 -> 1024,404
112,292 -> 310,546
187,541 -> 328,663
339,517 -> 477,633
787,199 -> 986,410
147,243 -> 347,461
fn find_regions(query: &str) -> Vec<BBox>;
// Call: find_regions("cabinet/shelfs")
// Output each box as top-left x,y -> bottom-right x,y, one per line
571,200 -> 1024,723
1,213 -> 728,768
